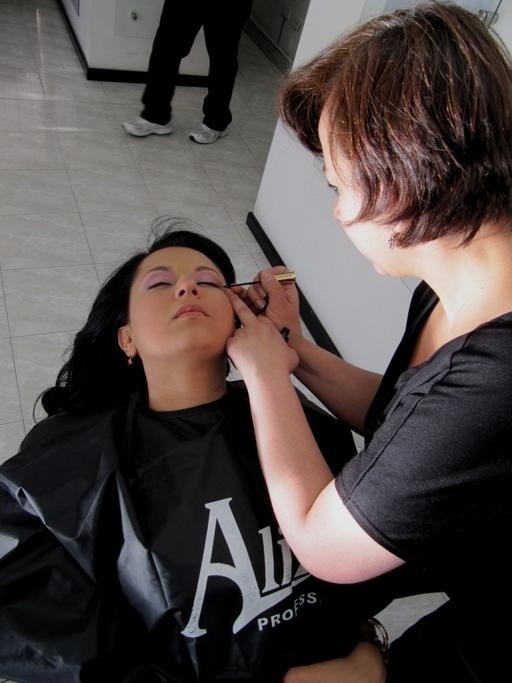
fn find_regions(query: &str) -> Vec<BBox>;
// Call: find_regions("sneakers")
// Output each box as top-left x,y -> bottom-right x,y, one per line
121,116 -> 174,138
190,123 -> 231,144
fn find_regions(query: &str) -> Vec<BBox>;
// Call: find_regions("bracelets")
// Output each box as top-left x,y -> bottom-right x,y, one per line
368,617 -> 390,668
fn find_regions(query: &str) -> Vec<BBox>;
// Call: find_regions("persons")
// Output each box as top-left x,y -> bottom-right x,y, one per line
223,0 -> 511,683
120,0 -> 247,147
0,212 -> 390,682
